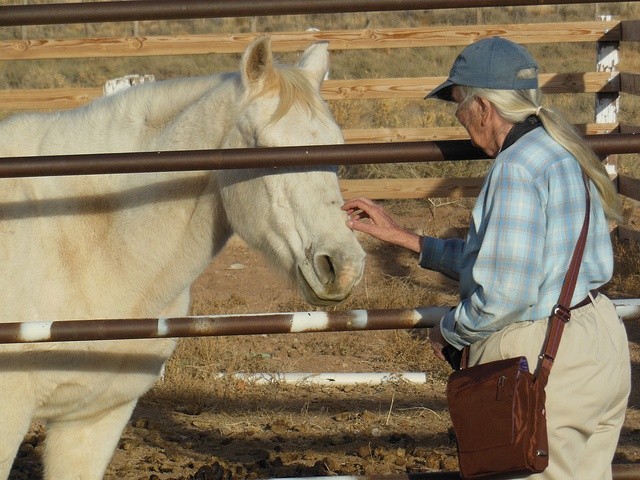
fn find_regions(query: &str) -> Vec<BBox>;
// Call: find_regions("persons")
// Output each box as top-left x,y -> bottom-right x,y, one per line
341,35 -> 632,479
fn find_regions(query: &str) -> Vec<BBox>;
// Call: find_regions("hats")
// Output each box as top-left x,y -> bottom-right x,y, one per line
423,35 -> 539,102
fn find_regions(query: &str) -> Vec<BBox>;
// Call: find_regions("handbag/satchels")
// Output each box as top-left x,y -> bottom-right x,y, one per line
447,356 -> 548,479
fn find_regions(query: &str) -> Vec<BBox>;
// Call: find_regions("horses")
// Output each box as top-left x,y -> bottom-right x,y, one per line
0,36 -> 366,480
